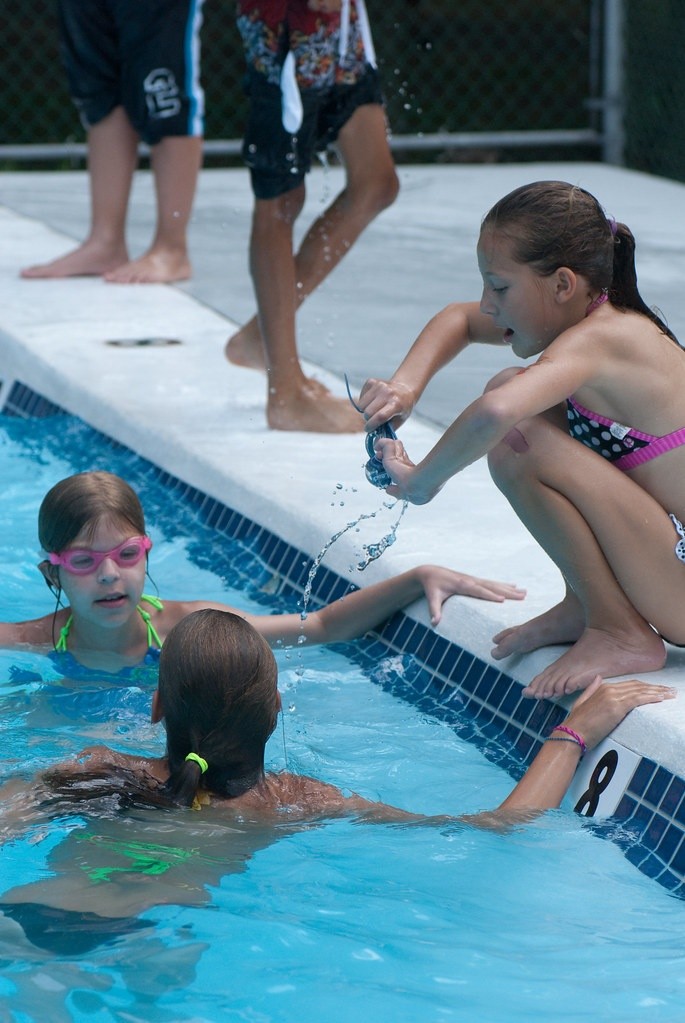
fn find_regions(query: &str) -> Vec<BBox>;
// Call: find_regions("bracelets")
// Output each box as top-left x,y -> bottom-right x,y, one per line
551,724 -> 586,755
542,737 -> 587,751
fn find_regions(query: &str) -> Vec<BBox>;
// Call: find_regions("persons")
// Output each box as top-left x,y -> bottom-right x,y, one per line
215,0 -> 406,436
0,605 -> 681,1023
0,468 -> 529,707
352,185 -> 685,698
3,0 -> 218,287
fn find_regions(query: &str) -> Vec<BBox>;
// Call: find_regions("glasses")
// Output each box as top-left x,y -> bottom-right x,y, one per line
344,373 -> 397,488
37,535 -> 152,576
